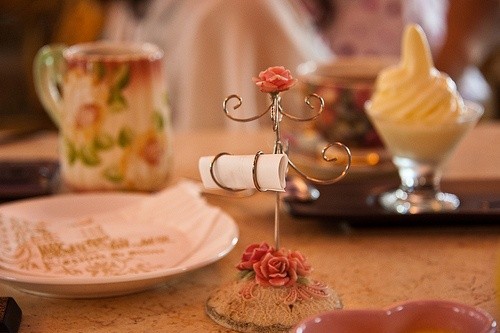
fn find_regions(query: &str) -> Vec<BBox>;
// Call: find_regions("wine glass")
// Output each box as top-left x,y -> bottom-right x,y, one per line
363,99 -> 485,211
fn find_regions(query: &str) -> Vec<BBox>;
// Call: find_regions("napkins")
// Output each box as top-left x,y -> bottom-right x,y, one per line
198,154 -> 289,192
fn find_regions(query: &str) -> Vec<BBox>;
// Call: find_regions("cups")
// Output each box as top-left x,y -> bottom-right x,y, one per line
33,39 -> 174,193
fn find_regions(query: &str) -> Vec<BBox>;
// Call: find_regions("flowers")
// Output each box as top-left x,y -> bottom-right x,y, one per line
252,64 -> 296,94
235,241 -> 315,289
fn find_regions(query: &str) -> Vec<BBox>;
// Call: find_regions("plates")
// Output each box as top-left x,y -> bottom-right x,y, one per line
282,176 -> 500,228
287,300 -> 497,333
0,191 -> 240,298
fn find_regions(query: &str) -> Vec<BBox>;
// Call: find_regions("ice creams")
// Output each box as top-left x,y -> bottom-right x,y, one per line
363,23 -> 485,171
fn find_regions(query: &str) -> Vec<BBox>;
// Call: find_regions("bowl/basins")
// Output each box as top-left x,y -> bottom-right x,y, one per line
297,55 -> 401,174
